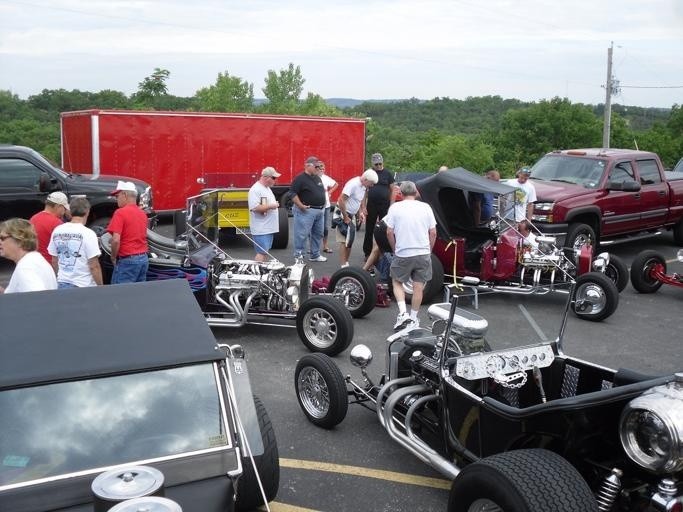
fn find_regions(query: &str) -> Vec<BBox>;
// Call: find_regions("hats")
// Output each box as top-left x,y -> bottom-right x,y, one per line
109,180 -> 137,198
262,166 -> 282,178
521,165 -> 531,176
46,191 -> 70,212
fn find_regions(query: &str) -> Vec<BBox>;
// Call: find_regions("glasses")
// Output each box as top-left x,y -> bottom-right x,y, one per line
373,163 -> 382,166
0,234 -> 11,241
270,175 -> 276,180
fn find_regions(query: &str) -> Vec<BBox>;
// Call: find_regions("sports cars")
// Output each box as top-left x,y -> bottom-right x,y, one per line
175,172 -> 291,252
325,168 -> 629,322
288,279 -> 683,511
88,223 -> 377,355
628,247 -> 683,293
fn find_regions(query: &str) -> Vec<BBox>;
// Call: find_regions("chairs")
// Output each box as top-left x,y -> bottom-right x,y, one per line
100,232 -> 114,255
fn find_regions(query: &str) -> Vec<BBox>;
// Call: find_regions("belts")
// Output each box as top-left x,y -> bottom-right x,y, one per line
304,203 -> 324,209
117,252 -> 148,261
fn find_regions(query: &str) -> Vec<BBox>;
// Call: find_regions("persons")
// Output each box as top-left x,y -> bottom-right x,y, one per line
518,220 -> 539,251
0,180 -> 149,294
247,152 -> 440,332
469,171 -> 500,230
504,166 -> 537,229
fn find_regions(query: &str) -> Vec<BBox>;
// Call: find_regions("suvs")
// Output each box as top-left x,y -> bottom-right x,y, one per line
0,145 -> 158,234
0,277 -> 281,512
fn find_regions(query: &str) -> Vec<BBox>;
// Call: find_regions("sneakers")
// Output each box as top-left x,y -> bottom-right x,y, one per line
322,247 -> 377,277
392,312 -> 412,332
406,315 -> 420,330
309,255 -> 328,262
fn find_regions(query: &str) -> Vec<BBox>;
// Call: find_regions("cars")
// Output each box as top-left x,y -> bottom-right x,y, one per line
673,156 -> 683,173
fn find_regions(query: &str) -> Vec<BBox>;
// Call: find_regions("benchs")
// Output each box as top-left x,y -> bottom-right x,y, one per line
483,366 -> 682,419
440,187 -> 494,274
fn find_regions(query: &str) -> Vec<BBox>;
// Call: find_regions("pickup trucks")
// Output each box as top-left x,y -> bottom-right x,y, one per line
502,147 -> 683,261
